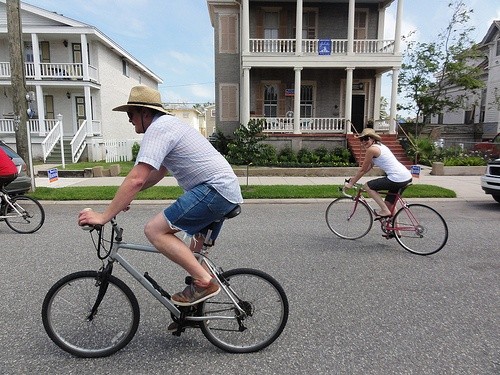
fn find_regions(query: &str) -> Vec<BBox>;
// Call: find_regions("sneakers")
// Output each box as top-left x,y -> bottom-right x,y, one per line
167,315 -> 210,332
170,278 -> 221,306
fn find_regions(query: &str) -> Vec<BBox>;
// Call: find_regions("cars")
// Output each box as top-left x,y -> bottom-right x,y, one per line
480,157 -> 500,202
0,136 -> 31,194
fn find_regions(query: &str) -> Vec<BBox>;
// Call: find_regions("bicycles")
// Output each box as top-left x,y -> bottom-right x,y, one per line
42,207 -> 290,358
0,178 -> 46,234
324,178 -> 449,256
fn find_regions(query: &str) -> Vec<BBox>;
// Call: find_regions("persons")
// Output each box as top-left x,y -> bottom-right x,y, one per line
0,145 -> 18,222
345,128 -> 413,238
79,84 -> 244,306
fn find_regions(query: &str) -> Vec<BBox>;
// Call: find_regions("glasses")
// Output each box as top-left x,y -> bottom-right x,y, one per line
127,110 -> 137,119
359,136 -> 370,142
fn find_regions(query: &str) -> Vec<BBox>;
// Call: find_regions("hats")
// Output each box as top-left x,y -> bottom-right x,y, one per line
112,86 -> 174,116
354,128 -> 381,141
